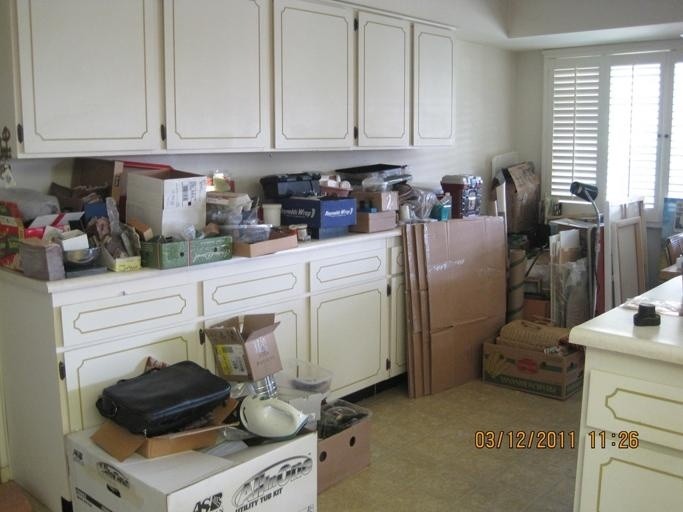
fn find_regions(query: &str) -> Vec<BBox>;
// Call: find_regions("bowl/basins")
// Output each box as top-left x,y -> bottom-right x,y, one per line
61,245 -> 101,268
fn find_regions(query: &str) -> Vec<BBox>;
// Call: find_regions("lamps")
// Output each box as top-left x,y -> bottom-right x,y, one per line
570,181 -> 601,317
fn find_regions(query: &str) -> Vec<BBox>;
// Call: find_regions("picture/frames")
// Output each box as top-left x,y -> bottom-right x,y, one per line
625,200 -> 650,291
610,216 -> 647,306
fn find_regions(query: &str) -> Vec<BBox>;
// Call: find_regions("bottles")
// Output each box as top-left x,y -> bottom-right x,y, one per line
435,190 -> 451,219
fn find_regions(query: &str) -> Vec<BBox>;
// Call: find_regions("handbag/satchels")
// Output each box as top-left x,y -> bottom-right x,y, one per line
95,361 -> 232,438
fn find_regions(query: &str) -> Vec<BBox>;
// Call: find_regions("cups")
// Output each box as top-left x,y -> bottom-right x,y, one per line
398,205 -> 411,221
289,224 -> 307,242
261,204 -> 282,226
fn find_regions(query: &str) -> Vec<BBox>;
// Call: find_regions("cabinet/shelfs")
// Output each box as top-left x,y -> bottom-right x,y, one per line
0,262 -> 204,511
390,245 -> 407,378
205,251 -> 306,380
573,346 -> 683,512
0,0 -> 459,160
306,239 -> 387,405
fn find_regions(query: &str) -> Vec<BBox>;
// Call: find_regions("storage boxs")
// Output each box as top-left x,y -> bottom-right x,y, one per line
440,173 -> 483,219
